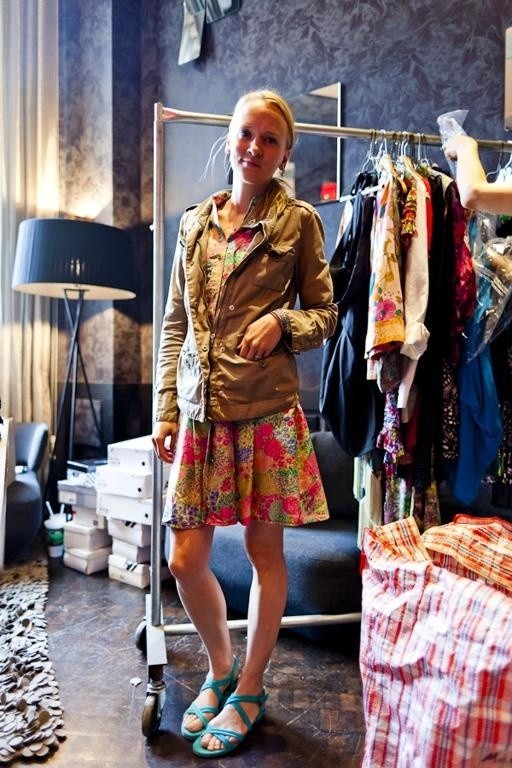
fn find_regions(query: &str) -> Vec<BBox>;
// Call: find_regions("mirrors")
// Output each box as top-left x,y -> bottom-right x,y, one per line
270,82 -> 339,205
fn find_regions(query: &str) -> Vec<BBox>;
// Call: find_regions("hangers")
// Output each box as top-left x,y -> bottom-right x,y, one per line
339,129 -> 512,202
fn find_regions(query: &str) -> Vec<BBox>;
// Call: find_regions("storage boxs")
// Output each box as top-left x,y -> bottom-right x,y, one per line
57,435 -> 171,589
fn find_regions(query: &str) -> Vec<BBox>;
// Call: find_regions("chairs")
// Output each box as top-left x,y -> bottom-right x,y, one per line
164,431 -> 365,641
0,422 -> 48,565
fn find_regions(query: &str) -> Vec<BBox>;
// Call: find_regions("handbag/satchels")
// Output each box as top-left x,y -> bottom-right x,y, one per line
358,514 -> 511,767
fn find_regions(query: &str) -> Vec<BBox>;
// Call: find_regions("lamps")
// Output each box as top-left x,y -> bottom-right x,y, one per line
11,218 -> 136,507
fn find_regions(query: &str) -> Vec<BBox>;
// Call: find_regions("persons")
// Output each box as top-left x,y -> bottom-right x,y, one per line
150,84 -> 344,762
440,134 -> 511,219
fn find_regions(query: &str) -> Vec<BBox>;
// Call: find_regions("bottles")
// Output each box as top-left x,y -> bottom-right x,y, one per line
45,520 -> 63,559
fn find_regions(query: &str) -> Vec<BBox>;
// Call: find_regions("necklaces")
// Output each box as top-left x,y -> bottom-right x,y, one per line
220,209 -> 244,231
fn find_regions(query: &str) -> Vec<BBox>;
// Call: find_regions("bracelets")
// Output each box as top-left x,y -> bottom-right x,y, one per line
268,311 -> 282,328
272,307 -> 294,339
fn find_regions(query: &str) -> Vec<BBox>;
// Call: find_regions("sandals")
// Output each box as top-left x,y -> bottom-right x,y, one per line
181,655 -> 268,758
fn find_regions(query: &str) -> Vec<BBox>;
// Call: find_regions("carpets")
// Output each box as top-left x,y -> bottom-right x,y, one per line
0,544 -> 67,763
